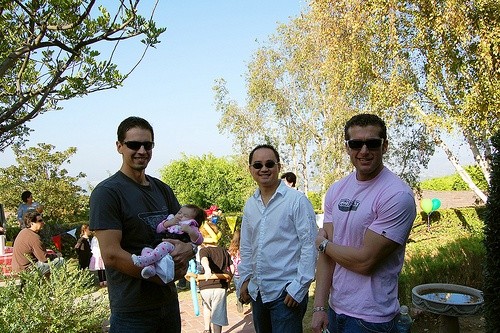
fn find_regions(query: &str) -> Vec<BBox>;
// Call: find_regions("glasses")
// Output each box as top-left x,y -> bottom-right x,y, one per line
347,138 -> 384,150
251,160 -> 278,169
122,141 -> 154,151
36,219 -> 43,222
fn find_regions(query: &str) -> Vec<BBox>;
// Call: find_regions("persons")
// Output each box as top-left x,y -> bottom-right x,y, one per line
131,204 -> 208,278
0,203 -> 7,255
227,229 -> 253,313
280,171 -> 297,189
310,114 -> 417,333
199,223 -> 235,333
34,206 -> 44,213
236,145 -> 318,333
89,116 -> 198,333
88,231 -> 107,287
18,190 -> 43,235
74,224 -> 93,270
12,209 -> 65,281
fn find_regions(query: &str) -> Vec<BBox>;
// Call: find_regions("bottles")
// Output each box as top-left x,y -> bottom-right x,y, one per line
397,305 -> 413,333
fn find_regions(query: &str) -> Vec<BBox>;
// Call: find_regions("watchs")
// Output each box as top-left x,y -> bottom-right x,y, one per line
187,241 -> 198,255
319,239 -> 329,256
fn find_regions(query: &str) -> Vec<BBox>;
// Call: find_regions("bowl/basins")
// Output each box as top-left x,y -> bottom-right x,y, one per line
412,283 -> 484,316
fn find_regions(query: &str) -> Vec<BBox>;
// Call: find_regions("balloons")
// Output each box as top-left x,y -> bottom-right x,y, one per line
420,198 -> 441,216
189,259 -> 200,316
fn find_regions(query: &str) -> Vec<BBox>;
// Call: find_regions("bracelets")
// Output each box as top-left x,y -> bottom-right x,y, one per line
312,306 -> 327,313
